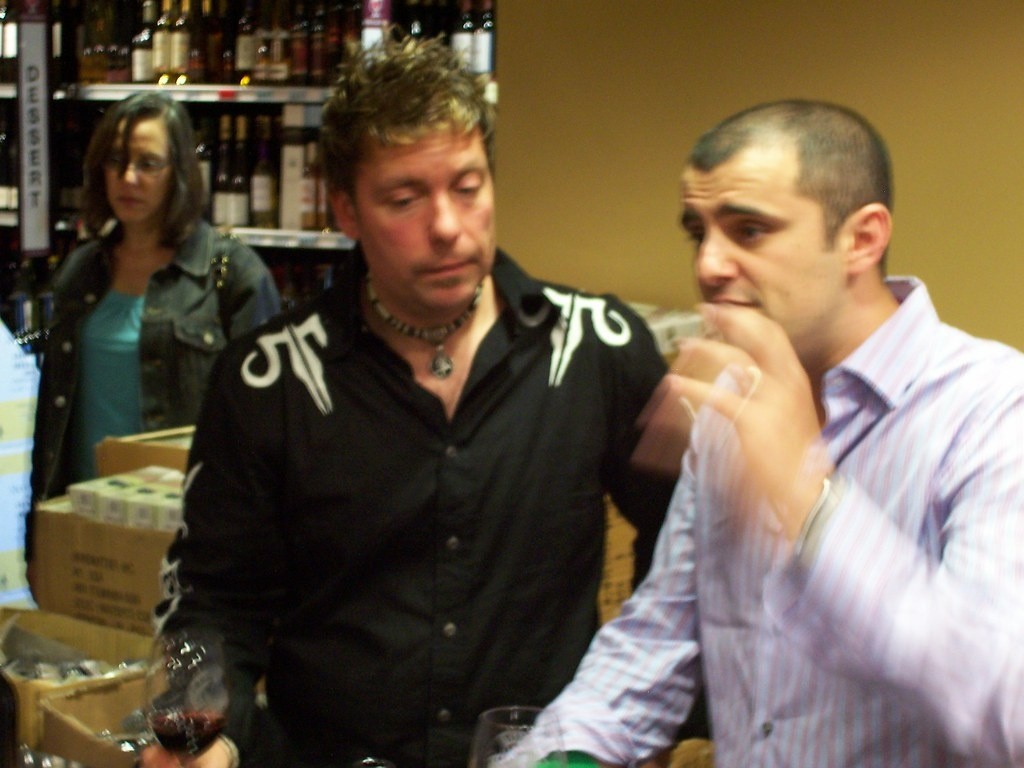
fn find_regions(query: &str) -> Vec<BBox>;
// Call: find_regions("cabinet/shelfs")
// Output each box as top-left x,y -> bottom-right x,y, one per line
0,84 -> 497,250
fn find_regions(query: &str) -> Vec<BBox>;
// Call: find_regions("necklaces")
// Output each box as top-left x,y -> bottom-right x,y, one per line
361,267 -> 484,377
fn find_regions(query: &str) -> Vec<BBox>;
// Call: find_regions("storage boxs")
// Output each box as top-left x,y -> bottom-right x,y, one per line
93,425 -> 197,477
0,607 -> 155,751
35,678 -> 146,768
34,495 -> 176,637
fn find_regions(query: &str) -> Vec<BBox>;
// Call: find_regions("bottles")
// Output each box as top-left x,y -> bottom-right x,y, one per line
13,256 -> 62,336
45,0 -> 494,84
0,0 -> 19,83
195,102 -> 330,230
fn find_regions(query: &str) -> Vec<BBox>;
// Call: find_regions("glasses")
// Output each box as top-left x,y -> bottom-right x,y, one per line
105,152 -> 175,176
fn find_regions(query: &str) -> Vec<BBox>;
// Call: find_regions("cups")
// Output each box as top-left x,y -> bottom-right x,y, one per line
468,706 -> 567,768
148,630 -> 230,766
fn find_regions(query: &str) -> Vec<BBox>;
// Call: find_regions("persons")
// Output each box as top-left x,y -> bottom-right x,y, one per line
25,90 -> 283,609
142,33 -> 715,768
485,100 -> 1024,768
